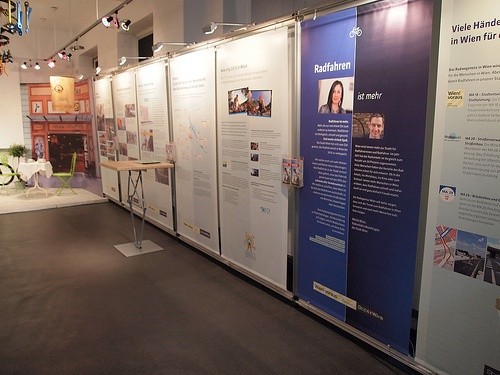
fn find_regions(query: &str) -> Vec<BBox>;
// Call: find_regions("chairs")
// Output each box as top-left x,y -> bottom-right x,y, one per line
52,152 -> 78,196
0,173 -> 21,193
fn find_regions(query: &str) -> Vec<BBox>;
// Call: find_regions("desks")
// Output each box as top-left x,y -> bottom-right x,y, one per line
18,160 -> 53,198
98,158 -> 174,257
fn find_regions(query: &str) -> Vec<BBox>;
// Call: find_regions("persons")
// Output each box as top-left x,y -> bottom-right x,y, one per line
250,141 -> 303,187
229,94 -> 268,117
318,80 -> 346,114
363,113 -> 384,140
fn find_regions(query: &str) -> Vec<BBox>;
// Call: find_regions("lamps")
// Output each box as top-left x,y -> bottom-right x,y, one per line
203,21 -> 256,34
21,48 -> 154,80
152,42 -> 198,52
101,8 -> 132,32
295,0 -> 358,23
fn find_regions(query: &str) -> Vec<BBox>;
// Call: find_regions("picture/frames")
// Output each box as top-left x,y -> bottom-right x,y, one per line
47,100 -> 66,114
31,100 -> 43,115
34,134 -> 45,160
